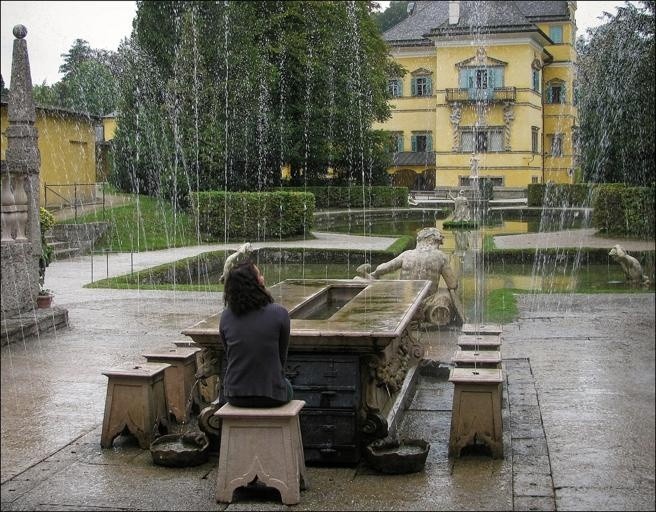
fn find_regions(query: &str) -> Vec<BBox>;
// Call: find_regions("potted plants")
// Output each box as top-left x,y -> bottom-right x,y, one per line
37,206 -> 56,310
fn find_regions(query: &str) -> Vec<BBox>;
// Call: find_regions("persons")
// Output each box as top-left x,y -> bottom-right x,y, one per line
469,152 -> 480,177
609,244 -> 642,287
373,228 -> 458,322
449,189 -> 471,222
219,264 -> 294,406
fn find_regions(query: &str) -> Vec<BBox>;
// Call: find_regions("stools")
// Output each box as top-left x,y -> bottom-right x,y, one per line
445,324 -> 508,460
215,398 -> 309,505
100,337 -> 215,448
